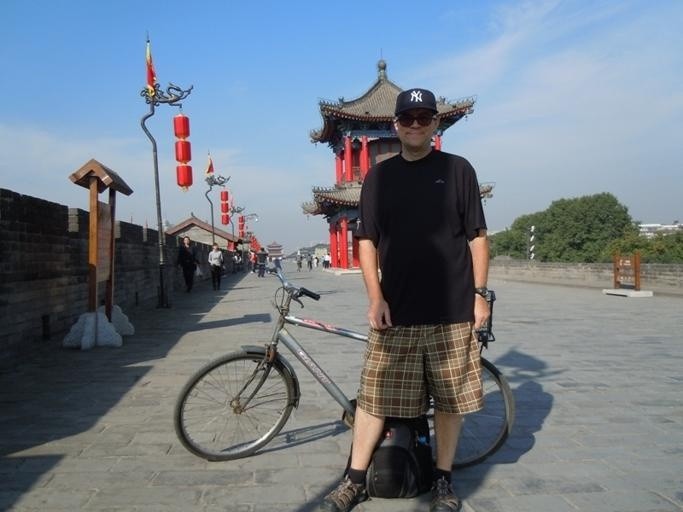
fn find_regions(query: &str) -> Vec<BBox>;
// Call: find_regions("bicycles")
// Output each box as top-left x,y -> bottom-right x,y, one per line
172,253 -> 513,471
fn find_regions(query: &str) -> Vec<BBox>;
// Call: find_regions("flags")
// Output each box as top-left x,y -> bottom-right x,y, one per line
145,40 -> 156,100
206,157 -> 214,174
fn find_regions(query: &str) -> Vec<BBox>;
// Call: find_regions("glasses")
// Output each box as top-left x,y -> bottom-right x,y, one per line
394,113 -> 437,127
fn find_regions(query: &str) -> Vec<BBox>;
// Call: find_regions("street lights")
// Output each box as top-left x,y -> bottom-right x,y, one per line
140,28 -> 194,305
203,147 -> 267,279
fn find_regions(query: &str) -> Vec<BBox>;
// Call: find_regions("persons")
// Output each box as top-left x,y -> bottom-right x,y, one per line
318,89 -> 492,512
292,248 -> 341,271
206,242 -> 223,291
176,236 -> 198,293
220,246 -> 281,278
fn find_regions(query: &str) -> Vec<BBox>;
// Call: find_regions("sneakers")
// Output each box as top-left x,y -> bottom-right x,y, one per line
431,480 -> 460,512
320,474 -> 367,511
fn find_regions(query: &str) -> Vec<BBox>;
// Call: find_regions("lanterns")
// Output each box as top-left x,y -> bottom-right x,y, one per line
220,213 -> 229,227
173,138 -> 191,163
238,215 -> 261,254
172,114 -> 190,140
220,190 -> 228,202
176,161 -> 192,192
220,202 -> 228,214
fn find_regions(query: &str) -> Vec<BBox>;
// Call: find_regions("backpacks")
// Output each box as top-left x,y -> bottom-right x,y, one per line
345,413 -> 433,498
220,262 -> 226,271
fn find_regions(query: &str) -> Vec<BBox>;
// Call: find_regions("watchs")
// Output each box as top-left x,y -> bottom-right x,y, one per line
474,287 -> 489,296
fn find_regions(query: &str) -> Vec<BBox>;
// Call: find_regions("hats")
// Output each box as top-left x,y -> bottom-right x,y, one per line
396,89 -> 439,114
